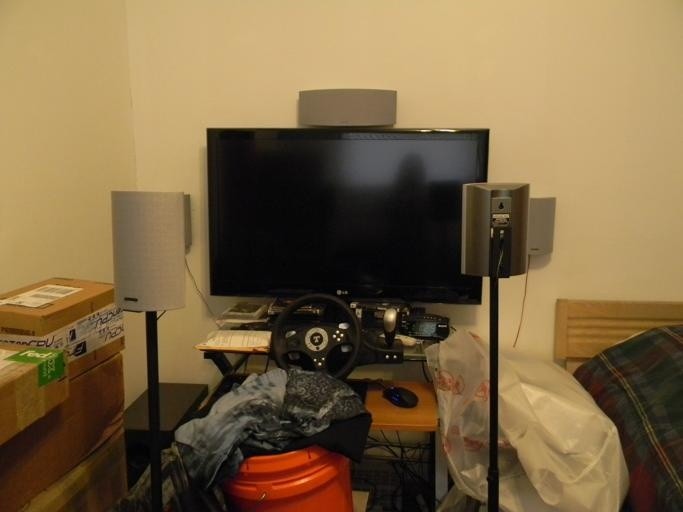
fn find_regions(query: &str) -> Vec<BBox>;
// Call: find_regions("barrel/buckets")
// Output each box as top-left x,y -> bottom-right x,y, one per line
229,447 -> 354,512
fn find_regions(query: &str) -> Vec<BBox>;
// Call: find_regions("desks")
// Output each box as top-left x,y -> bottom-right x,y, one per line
194,301 -> 451,511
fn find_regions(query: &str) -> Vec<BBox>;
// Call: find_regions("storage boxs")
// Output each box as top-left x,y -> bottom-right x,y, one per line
0,276 -> 130,512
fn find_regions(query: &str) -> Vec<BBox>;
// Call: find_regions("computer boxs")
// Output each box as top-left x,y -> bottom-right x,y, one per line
122,383 -> 210,491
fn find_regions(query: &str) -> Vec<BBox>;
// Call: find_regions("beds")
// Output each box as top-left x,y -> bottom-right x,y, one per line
554,299 -> 683,508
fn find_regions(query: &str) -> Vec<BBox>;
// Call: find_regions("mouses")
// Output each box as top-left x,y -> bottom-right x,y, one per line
383,385 -> 420,408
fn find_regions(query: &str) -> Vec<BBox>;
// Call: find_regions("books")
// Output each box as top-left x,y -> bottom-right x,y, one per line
216,301 -> 319,331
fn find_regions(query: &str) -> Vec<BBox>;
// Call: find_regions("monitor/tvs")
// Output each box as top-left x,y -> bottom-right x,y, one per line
205,125 -> 490,306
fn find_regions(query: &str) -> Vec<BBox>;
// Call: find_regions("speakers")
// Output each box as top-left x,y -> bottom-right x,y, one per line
110,190 -> 187,312
528,198 -> 556,258
298,88 -> 397,128
461,180 -> 528,280
